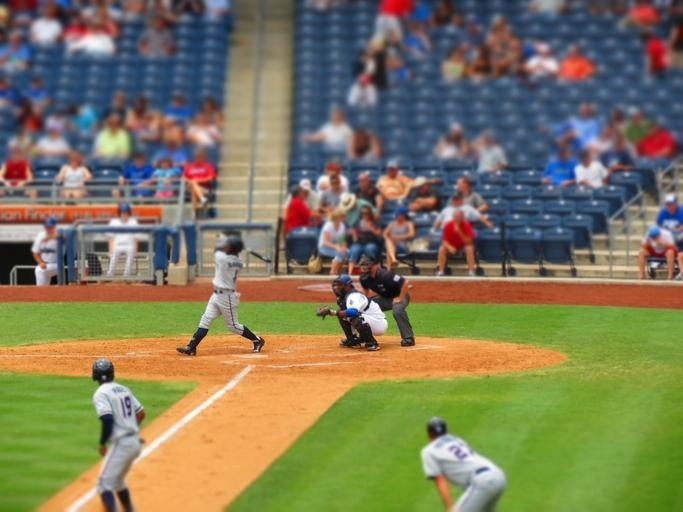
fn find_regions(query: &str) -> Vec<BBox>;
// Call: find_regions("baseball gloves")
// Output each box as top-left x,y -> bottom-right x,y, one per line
316,305 -> 330,316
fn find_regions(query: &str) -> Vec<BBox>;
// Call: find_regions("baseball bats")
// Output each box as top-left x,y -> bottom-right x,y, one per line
243,248 -> 271,263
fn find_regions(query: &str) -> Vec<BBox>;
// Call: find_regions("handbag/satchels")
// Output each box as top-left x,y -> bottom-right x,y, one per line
308,255 -> 321,273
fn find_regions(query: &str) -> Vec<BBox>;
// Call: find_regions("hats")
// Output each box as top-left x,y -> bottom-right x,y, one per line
665,194 -> 677,207
47,216 -> 59,229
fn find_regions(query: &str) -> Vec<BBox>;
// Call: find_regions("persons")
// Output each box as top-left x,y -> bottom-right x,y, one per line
315,275 -> 388,352
0,0 -> 683,293
89,357 -> 146,511
356,244 -> 417,347
174,231 -> 267,357
419,416 -> 509,512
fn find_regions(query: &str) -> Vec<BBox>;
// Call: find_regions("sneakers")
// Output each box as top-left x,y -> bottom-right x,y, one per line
253,337 -> 264,353
339,336 -> 379,351
400,338 -> 414,346
176,346 -> 195,356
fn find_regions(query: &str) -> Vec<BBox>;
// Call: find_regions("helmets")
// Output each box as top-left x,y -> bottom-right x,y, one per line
91,359 -> 113,381
426,418 -> 445,436
117,203 -> 132,218
331,274 -> 351,296
226,237 -> 242,251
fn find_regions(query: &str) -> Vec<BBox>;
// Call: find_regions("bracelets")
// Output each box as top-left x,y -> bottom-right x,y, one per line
330,309 -> 340,317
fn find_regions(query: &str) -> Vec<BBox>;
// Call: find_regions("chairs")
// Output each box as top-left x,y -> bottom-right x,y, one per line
0,1 -> 237,217
287,0 -> 681,277
